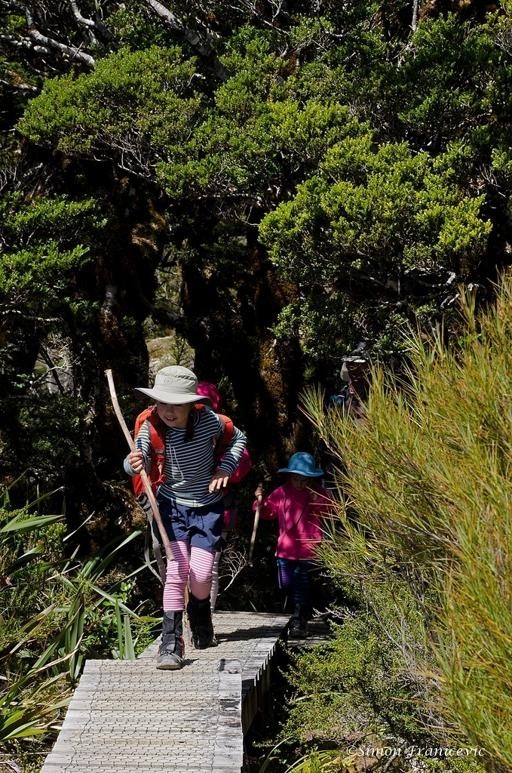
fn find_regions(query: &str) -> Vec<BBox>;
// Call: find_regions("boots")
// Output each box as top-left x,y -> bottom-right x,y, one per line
187,593 -> 213,647
291,602 -> 307,637
156,609 -> 182,669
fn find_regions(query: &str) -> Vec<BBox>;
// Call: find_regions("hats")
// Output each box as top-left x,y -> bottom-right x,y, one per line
196,381 -> 221,412
135,365 -> 211,404
278,451 -> 324,476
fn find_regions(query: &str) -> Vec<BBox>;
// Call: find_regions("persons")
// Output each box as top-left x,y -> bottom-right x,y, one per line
251,451 -> 342,640
122,363 -> 249,670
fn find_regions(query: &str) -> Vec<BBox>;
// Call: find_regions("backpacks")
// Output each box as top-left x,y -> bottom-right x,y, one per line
132,403 -> 234,497
